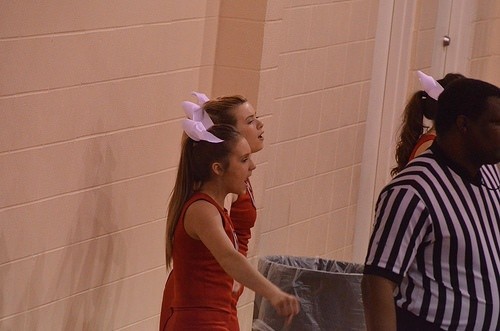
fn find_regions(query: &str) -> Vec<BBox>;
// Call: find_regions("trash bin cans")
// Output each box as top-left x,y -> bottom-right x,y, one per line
249,256 -> 367,331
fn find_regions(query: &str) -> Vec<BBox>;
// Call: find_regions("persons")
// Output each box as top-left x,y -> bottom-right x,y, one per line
360,78 -> 500,330
191,90 -> 264,331
159,100 -> 300,330
389,72 -> 467,178
